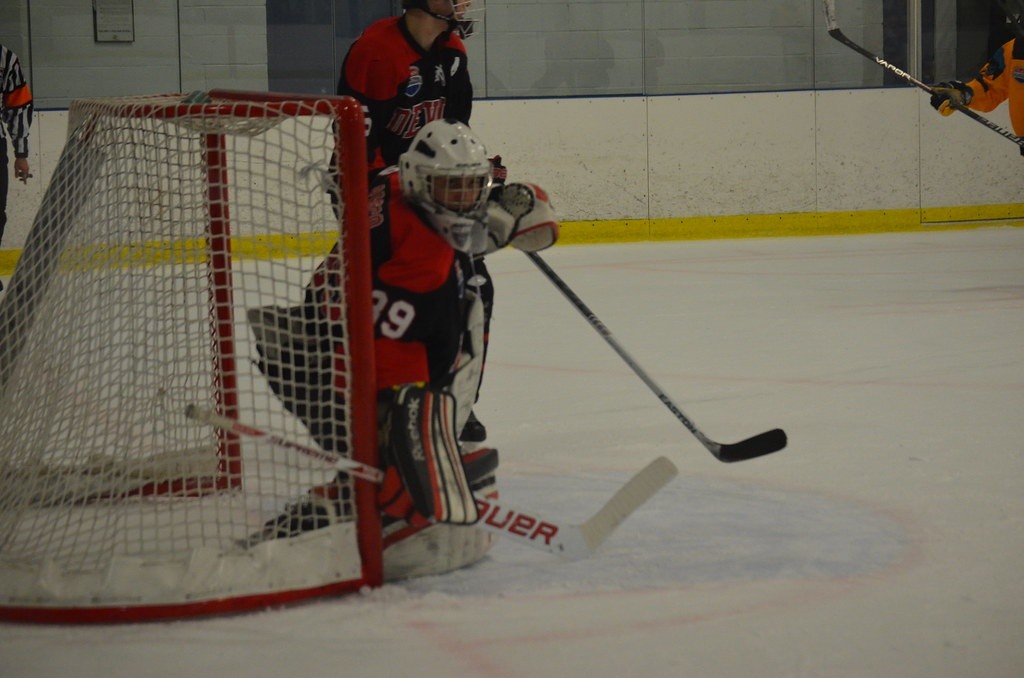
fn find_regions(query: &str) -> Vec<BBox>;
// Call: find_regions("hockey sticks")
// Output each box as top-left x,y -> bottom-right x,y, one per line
184,403 -> 680,561
523,249 -> 786,464
821,0 -> 1023,147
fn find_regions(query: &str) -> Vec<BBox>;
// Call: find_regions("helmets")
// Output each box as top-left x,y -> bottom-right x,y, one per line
398,120 -> 492,252
400,0 -> 484,40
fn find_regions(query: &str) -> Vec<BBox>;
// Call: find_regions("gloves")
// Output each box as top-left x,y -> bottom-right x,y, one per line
929,80 -> 975,116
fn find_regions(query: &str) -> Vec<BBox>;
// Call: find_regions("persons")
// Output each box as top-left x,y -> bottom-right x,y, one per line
0,44 -> 34,291
321,0 -> 485,217
303,116 -> 560,582
930,30 -> 1024,157
